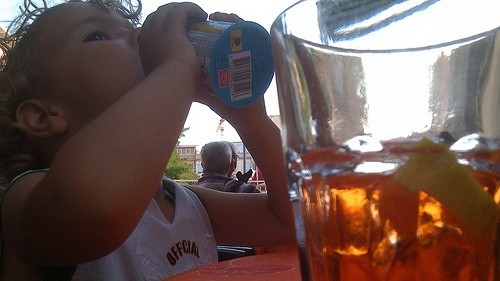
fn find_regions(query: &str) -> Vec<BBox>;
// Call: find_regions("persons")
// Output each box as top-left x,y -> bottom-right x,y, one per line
195,142 -> 261,192
0,0 -> 297,281
219,141 -> 253,183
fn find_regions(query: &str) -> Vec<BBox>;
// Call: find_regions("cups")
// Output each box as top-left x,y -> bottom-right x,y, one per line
138,10 -> 275,108
270,0 -> 500,281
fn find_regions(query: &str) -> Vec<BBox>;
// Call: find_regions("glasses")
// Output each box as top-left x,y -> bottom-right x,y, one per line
232,155 -> 239,161
